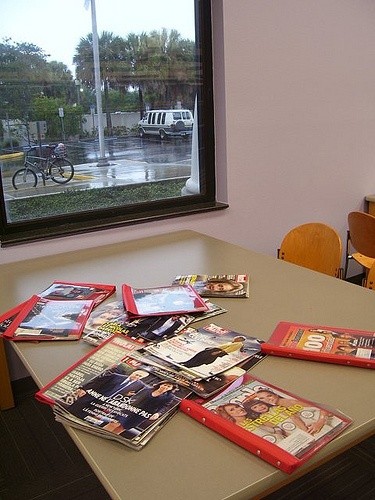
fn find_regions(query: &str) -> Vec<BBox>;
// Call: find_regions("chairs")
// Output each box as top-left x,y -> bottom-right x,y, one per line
342,211 -> 375,280
276,221 -> 345,280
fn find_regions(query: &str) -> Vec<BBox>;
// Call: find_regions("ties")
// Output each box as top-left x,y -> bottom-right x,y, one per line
107,380 -> 130,396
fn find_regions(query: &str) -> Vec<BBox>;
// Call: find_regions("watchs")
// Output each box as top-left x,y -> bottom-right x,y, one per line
320,415 -> 329,421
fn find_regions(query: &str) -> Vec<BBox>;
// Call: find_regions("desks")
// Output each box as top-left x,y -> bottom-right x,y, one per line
0,230 -> 375,500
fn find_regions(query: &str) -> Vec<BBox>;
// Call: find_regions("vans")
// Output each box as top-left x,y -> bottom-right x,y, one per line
137,109 -> 194,140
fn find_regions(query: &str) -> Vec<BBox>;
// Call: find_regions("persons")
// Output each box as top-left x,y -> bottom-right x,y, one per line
309,329 -> 359,356
101,380 -> 179,434
216,389 -> 342,445
180,336 -> 246,368
137,313 -> 182,343
86,300 -> 126,328
52,286 -> 82,296
193,277 -> 243,294
66,370 -> 149,421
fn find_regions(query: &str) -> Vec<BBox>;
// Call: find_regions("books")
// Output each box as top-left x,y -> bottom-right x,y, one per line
261,321 -> 375,369
180,373 -> 353,475
0,274 -> 268,451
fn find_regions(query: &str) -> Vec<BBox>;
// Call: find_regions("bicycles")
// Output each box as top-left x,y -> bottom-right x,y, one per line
12,143 -> 75,191
